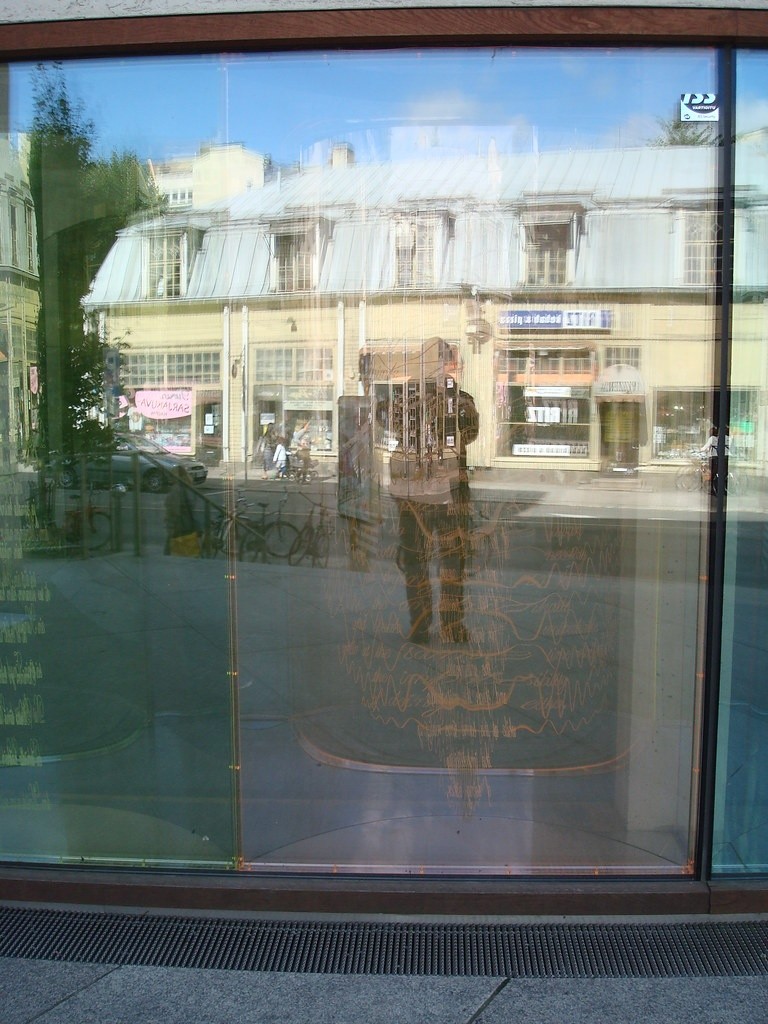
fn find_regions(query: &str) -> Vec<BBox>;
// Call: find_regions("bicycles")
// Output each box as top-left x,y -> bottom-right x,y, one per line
394,507 -> 493,578
678,450 -> 748,496
25,480 -> 113,552
198,487 -> 336,570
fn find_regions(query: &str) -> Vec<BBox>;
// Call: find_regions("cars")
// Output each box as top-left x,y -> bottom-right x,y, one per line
40,431 -> 209,490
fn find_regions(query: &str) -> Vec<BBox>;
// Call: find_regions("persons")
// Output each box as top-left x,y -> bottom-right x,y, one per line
700,427 -> 729,496
163,465 -> 204,559
293,439 -> 311,485
272,437 -> 292,479
373,337 -> 480,641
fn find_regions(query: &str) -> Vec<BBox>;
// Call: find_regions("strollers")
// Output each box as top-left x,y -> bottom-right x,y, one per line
289,452 -> 319,482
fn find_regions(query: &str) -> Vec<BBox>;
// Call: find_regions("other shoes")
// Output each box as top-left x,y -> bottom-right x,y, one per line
276,476 -> 282,481
438,606 -> 470,644
408,614 -> 433,645
262,475 -> 269,480
